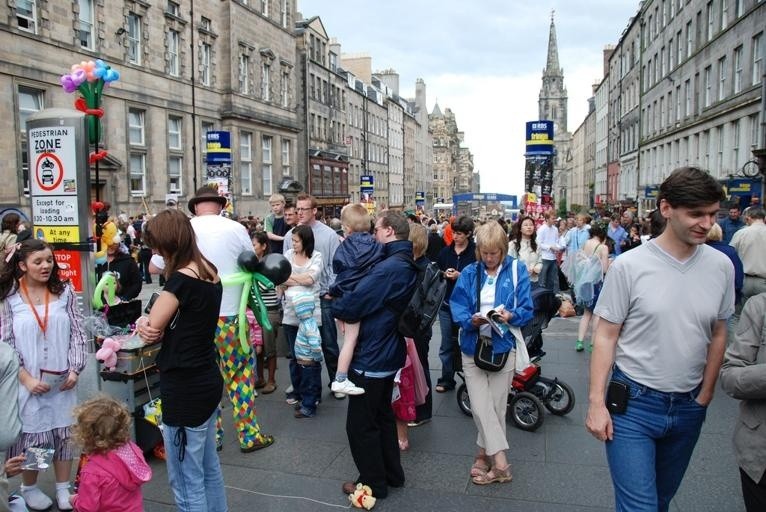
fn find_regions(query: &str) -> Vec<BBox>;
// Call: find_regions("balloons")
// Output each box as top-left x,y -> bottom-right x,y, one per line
92,202 -> 104,213
89,151 -> 108,164
93,273 -> 116,310
60,58 -> 119,145
95,221 -> 116,257
221,250 -> 292,354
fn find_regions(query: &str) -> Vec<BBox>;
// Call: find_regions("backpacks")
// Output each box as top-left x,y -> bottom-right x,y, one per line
377,253 -> 449,346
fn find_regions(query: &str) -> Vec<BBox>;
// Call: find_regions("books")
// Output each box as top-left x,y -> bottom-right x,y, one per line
475,304 -> 509,339
20,447 -> 56,472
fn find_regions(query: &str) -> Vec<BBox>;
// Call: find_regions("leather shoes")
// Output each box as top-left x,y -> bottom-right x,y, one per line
294,402 -> 302,410
342,481 -> 357,494
294,410 -> 306,418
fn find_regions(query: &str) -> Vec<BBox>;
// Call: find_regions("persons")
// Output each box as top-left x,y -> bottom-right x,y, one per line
69,397 -> 152,512
0,212 -> 20,289
622,223 -> 642,252
330,203 -> 382,397
328,218 -> 343,239
290,291 -> 324,417
535,211 -> 559,316
555,220 -> 569,292
265,192 -> 286,255
705,221 -> 743,355
284,202 -> 299,230
114,215 -> 137,261
590,209 -> 611,225
621,210 -> 634,228
450,220 -> 534,485
137,208 -> 227,511
727,206 -> 766,347
141,212 -> 153,283
407,223 -> 433,428
276,225 -> 323,407
606,214 -> 626,264
717,207 -> 741,244
342,209 -> 415,498
0,240 -> 88,511
558,213 -> 592,315
283,195 -> 346,404
585,168 -> 736,512
95,235 -> 141,307
0,339 -> 23,512
125,217 -> 136,245
185,184 -> 274,452
249,231 -> 281,392
564,217 -> 575,245
720,292 -> 766,512
133,215 -> 143,252
505,216 -> 546,357
575,225 -> 608,353
244,306 -> 264,396
435,217 -> 478,393
426,224 -> 443,262
407,213 -> 440,232
0,452 -> 26,478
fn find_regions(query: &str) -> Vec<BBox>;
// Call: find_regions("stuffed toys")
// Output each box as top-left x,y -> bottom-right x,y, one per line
348,483 -> 377,511
94,337 -> 120,374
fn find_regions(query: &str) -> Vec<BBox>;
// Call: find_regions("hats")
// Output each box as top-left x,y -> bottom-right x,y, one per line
188,187 -> 227,216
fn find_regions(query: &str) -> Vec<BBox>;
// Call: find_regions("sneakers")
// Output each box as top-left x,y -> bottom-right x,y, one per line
331,378 -> 365,396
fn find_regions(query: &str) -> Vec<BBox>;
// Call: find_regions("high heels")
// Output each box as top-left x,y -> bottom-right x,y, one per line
398,438 -> 409,451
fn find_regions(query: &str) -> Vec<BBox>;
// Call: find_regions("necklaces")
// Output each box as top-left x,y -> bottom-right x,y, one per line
35,296 -> 41,303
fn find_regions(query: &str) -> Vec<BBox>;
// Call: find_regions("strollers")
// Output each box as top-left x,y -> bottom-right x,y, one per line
457,290 -> 574,432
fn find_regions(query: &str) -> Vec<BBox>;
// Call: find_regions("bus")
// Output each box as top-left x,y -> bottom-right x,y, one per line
644,176 -> 762,238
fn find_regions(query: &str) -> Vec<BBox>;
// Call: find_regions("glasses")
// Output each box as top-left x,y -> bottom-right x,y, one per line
295,207 -> 311,212
374,225 -> 388,236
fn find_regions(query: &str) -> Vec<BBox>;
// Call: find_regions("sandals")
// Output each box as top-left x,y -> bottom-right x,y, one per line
240,434 -> 274,453
286,391 -> 299,406
435,383 -> 449,393
254,381 -> 265,389
261,382 -> 276,394
216,446 -> 223,451
472,464 -> 513,484
470,454 -> 491,477
